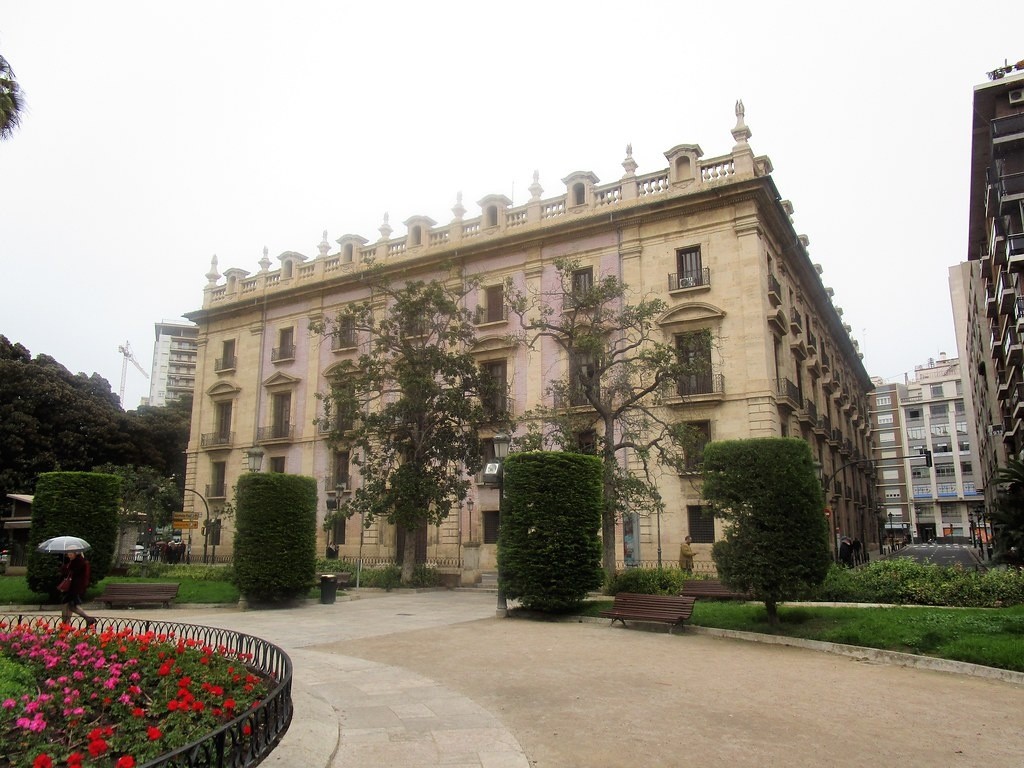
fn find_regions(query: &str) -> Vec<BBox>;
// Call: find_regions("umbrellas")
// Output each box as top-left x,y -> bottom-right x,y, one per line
36,536 -> 91,566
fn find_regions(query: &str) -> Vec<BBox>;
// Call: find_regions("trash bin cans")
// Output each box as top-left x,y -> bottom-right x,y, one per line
320,575 -> 338,604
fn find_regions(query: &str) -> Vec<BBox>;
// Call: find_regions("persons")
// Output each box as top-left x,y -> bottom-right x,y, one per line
853,537 -> 861,566
839,536 -> 854,569
679,536 -> 697,577
62,545 -> 98,633
150,539 -> 186,564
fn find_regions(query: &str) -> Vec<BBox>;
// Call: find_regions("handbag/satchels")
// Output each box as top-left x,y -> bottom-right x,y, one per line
57,570 -> 73,592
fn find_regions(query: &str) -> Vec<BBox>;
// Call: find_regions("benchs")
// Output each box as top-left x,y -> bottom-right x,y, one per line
599,592 -> 696,634
94,583 -> 182,608
316,571 -> 354,590
684,580 -> 752,599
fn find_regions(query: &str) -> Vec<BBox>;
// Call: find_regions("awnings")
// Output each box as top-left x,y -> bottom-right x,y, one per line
885,521 -> 911,529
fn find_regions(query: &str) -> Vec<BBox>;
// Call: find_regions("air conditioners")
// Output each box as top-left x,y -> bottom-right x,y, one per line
680,277 -> 695,287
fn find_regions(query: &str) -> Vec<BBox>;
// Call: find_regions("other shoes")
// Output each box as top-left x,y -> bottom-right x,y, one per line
85,617 -> 97,630
